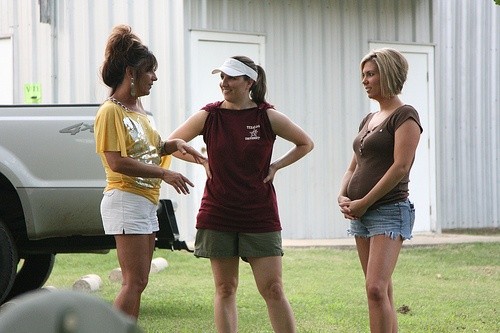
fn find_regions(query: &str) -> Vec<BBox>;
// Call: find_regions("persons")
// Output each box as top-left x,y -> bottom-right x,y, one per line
337,47 -> 423,332
93,24 -> 206,322
165,56 -> 314,333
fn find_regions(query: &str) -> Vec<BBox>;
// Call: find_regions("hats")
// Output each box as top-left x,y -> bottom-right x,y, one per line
212,57 -> 258,82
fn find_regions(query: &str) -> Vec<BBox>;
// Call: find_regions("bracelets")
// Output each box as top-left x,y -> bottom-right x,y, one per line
162,142 -> 172,155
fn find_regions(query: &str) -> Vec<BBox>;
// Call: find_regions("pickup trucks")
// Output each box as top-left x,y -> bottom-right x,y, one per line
0,104 -> 194,308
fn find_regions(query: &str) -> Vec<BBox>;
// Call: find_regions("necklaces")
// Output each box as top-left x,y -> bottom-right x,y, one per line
107,96 -> 133,111
